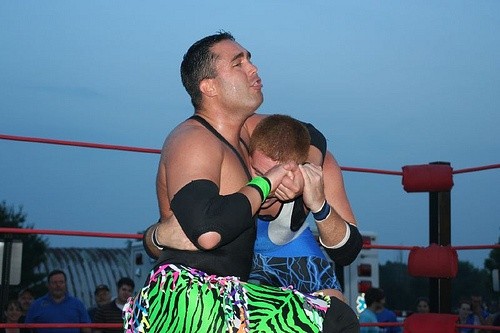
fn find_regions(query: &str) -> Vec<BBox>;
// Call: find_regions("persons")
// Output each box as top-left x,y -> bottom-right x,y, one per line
359,286 -> 499,332
0,270 -> 135,333
141,115 -> 363,303
125,31 -> 363,333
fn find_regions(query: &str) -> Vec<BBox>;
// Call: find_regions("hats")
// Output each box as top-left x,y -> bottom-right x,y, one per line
94,285 -> 110,293
364,287 -> 385,304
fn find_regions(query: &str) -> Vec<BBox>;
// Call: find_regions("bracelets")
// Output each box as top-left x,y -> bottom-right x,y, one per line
151,225 -> 165,251
312,198 -> 331,224
